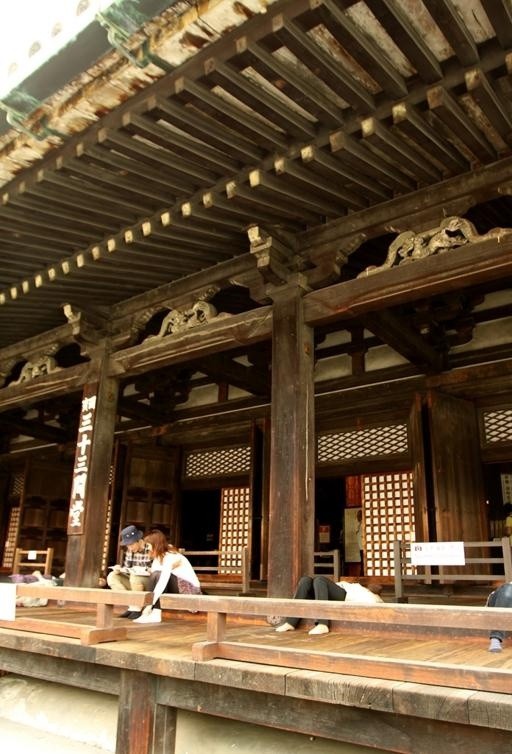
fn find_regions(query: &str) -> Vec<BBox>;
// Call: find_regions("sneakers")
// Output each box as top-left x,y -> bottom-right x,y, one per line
489,639 -> 502,652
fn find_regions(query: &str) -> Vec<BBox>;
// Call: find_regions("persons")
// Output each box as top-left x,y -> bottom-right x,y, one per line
8,570 -> 106,607
106,524 -> 153,619
275,575 -> 384,635
355,510 -> 364,576
484,582 -> 512,652
132,528 -> 203,627
502,502 -> 512,548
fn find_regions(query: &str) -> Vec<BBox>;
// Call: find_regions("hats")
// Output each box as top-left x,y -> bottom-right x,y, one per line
121,526 -> 143,546
